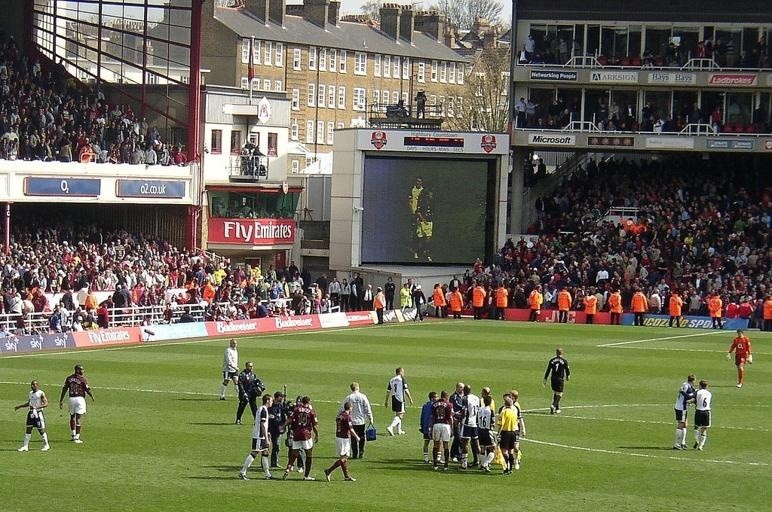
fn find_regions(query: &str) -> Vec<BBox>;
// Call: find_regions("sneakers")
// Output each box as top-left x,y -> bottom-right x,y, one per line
344,475 -> 357,482
235,419 -> 242,425
736,383 -> 742,388
398,430 -> 406,435
74,439 -> 84,444
238,465 -> 315,481
68,435 -> 75,441
324,469 -> 332,482
424,457 -> 520,476
550,404 -> 555,414
17,446 -> 29,452
673,440 -> 703,451
220,396 -> 225,400
386,426 -> 395,437
40,444 -> 51,452
555,409 -> 561,414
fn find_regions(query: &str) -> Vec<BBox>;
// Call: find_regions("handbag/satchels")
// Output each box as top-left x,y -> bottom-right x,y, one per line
366,422 -> 376,441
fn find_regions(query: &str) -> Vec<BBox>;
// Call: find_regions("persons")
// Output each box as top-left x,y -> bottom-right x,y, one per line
14,379 -> 50,452
673,374 -> 700,451
686,379 -> 713,452
543,348 -> 571,414
236,362 -> 527,481
59,364 -> 95,444
219,339 -> 240,401
726,328 -> 752,388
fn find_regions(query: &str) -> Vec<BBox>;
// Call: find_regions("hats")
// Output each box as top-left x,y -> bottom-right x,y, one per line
274,392 -> 287,398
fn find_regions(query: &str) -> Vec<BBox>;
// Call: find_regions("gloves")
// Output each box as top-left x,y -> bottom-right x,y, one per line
727,353 -> 732,359
566,375 -> 570,381
542,378 -> 547,389
747,355 -> 752,363
